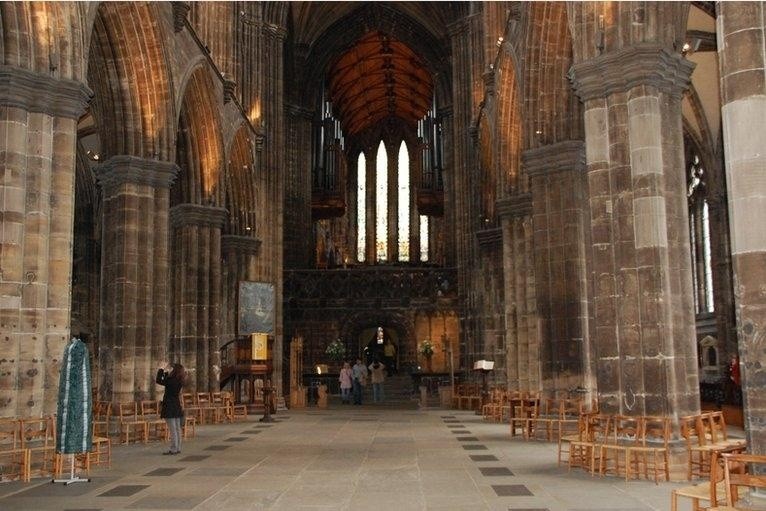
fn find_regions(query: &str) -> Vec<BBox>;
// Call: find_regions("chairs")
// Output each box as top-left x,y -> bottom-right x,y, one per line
0,387 -> 247,484
671,410 -> 765,510
450,385 -> 582,442
558,411 -> 671,485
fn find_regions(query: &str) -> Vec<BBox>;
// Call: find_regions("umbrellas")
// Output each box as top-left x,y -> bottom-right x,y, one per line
54,337 -> 94,480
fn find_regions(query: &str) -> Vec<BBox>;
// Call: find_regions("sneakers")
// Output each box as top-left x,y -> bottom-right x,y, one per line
161,450 -> 181,457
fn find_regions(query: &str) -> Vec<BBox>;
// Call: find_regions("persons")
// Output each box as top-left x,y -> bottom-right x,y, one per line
339,362 -> 354,405
349,358 -> 369,406
154,362 -> 187,457
384,338 -> 396,378
366,360 -> 386,403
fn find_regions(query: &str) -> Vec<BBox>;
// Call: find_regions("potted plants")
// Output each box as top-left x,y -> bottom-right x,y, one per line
325,336 -> 352,375
422,343 -> 436,373
